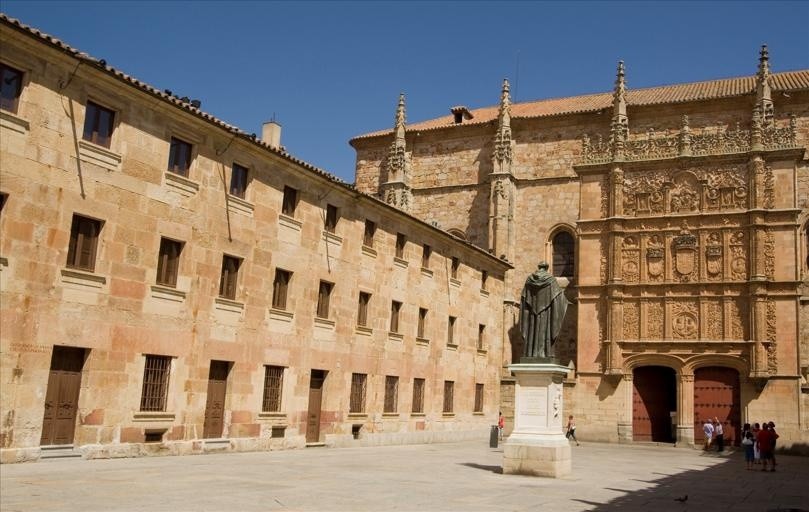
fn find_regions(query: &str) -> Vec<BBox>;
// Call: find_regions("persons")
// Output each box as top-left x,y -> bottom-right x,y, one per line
741,422 -> 779,472
499,412 -> 505,440
566,416 -> 580,446
518,261 -> 573,357
703,419 -> 714,451
714,416 -> 723,452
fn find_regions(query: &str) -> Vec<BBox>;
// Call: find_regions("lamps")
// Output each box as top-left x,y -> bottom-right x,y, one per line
216,133 -> 256,157
318,183 -> 356,200
59,59 -> 106,89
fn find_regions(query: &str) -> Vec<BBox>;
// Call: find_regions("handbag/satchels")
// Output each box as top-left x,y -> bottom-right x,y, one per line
740,432 -> 754,445
